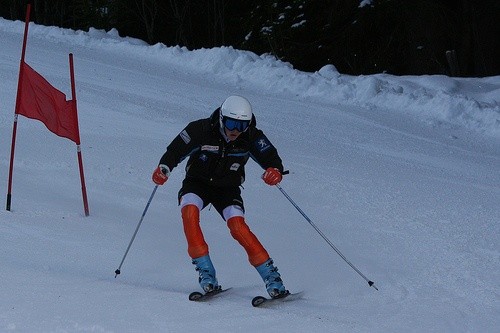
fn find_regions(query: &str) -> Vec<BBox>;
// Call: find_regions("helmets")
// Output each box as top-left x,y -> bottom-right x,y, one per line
220,97 -> 252,132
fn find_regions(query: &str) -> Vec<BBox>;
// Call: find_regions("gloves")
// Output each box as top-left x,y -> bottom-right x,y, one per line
263,167 -> 282,185
152,166 -> 168,185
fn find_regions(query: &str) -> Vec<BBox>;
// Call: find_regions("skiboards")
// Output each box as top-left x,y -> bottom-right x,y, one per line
188,287 -> 233,301
251,291 -> 305,307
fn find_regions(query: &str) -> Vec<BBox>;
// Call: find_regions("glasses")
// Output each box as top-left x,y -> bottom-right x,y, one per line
223,116 -> 250,132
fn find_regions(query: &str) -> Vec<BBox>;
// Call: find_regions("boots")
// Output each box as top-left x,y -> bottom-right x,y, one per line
253,258 -> 285,297
192,255 -> 218,293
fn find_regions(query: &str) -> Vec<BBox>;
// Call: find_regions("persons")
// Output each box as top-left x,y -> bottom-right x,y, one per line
152,94 -> 290,298
